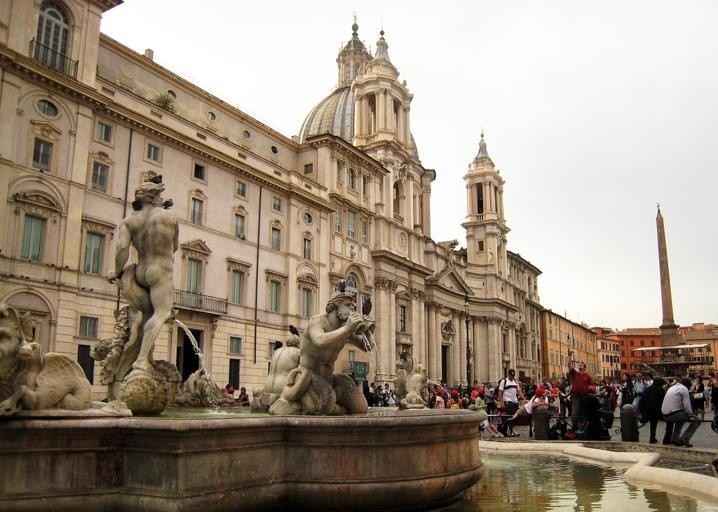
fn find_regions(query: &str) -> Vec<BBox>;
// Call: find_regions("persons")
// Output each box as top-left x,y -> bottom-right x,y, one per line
495,369 -> 718,447
365,380 -> 395,407
283,290 -> 375,414
263,333 -> 300,392
239,387 -> 250,406
424,380 -> 494,431
567,347 -> 596,430
109,181 -> 179,376
223,384 -> 235,399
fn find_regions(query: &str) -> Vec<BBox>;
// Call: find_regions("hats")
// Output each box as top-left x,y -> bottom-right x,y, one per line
509,369 -> 515,375
451,390 -> 458,396
536,388 -> 543,395
462,397 -> 470,406
551,381 -> 556,385
478,388 -> 485,394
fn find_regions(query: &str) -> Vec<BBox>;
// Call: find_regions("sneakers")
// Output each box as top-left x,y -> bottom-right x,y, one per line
492,429 -> 520,438
648,436 -> 693,448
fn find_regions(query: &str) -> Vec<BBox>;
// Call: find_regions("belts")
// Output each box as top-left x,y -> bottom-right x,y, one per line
667,410 -> 683,416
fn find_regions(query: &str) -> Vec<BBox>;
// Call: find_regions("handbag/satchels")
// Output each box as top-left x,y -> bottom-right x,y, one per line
551,420 -> 589,439
629,383 -> 637,397
694,392 -> 704,400
